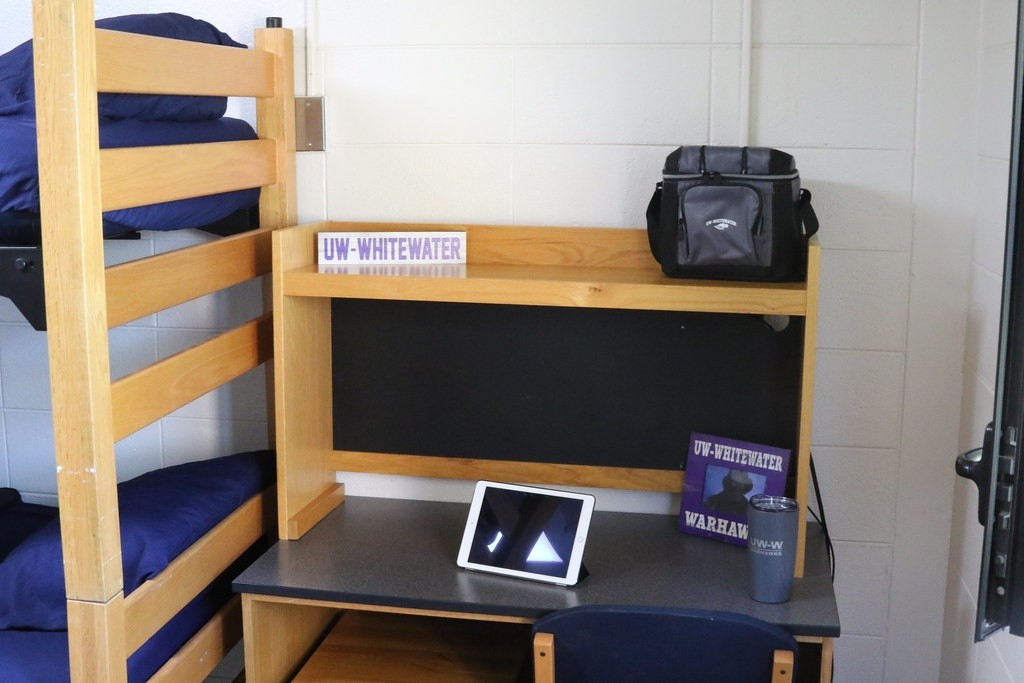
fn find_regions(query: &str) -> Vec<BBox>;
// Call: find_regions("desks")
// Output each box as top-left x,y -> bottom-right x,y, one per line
230,222 -> 842,683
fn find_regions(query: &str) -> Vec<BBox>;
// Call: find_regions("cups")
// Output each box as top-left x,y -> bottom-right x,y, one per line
745,495 -> 797,604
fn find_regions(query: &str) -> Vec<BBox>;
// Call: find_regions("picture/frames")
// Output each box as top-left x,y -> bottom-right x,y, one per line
677,431 -> 791,546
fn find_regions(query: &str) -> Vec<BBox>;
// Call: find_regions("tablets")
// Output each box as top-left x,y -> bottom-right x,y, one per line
457,480 -> 596,585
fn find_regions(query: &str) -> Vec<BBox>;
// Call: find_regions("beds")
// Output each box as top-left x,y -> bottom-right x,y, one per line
0,0 -> 304,683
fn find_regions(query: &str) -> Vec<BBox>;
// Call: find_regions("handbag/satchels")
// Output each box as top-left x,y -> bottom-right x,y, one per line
645,145 -> 818,283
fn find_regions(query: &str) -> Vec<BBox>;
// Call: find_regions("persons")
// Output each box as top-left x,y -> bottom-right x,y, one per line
705,468 -> 754,517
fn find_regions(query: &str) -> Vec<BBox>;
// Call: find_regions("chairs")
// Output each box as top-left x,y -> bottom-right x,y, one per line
527,604 -> 801,683
